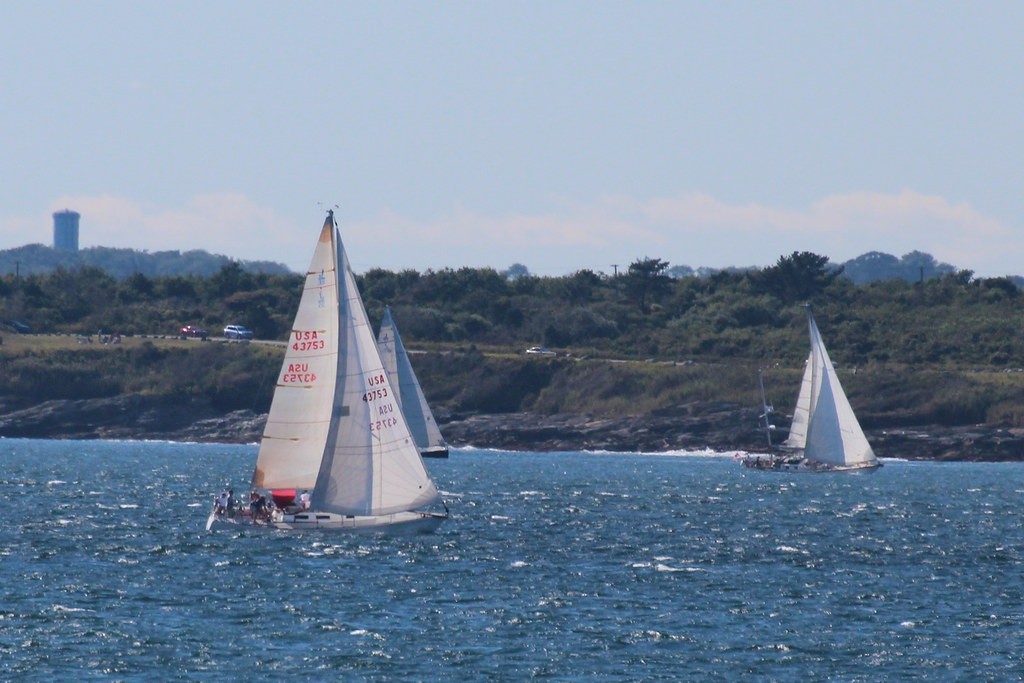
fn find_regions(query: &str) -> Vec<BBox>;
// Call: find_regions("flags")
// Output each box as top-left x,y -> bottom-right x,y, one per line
734,454 -> 742,460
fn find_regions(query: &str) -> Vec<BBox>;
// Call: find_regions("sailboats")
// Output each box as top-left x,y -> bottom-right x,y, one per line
374,303 -> 451,460
729,303 -> 885,475
206,202 -> 446,536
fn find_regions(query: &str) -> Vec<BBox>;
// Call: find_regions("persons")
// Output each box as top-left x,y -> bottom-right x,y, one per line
744,453 -> 807,469
213,488 -> 310,524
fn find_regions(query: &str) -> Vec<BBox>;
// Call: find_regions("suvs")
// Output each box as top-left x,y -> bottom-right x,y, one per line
223,324 -> 254,341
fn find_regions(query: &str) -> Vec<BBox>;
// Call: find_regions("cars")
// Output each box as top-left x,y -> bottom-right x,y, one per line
179,323 -> 209,339
525,345 -> 557,357
